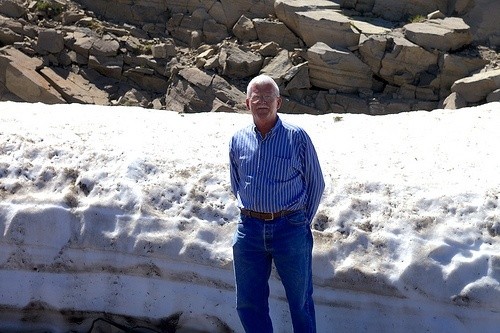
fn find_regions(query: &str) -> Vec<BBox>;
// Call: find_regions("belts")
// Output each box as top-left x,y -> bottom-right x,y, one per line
241,206 -> 305,221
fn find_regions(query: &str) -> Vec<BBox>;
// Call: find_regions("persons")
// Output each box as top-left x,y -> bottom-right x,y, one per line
227,72 -> 329,333
